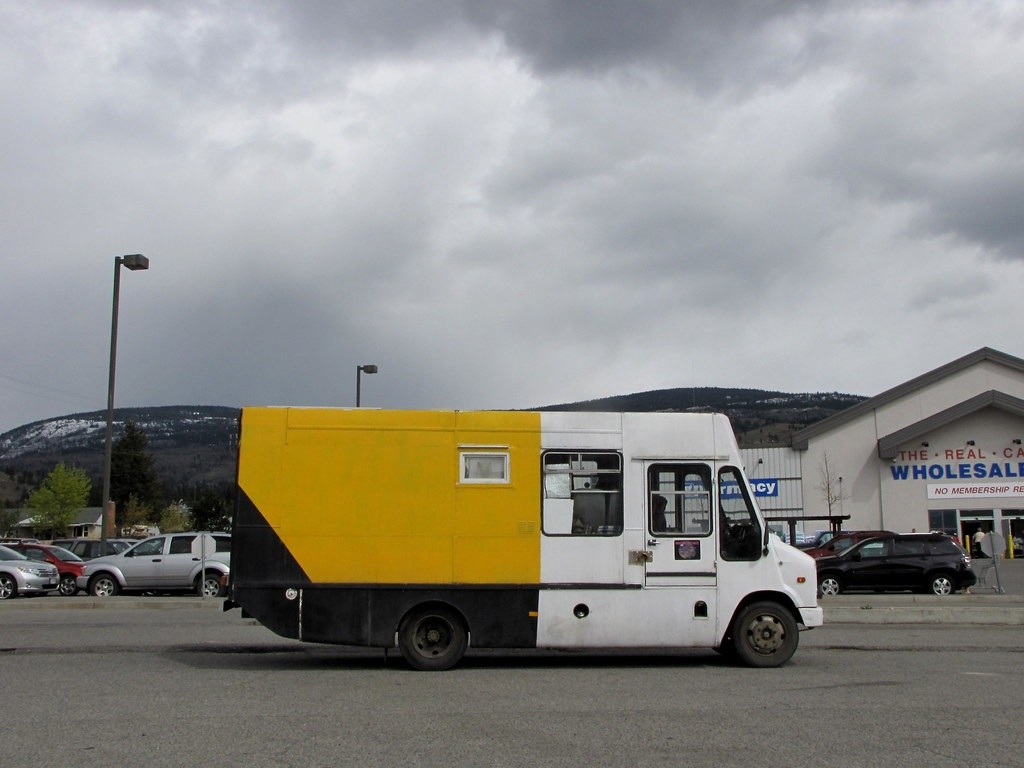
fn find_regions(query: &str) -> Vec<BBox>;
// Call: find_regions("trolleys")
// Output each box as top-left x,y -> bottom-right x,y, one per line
969,556 -> 1001,593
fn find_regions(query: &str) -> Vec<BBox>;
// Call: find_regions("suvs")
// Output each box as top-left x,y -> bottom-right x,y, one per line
76,531 -> 232,598
795,530 -> 897,560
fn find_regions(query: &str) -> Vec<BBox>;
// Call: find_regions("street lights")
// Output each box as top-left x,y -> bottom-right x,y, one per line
357,365 -> 379,408
99,255 -> 149,554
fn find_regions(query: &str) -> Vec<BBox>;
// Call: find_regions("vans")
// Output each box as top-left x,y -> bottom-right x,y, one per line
1,537 -> 39,543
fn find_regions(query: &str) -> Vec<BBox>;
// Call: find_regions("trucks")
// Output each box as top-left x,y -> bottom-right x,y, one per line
223,403 -> 823,675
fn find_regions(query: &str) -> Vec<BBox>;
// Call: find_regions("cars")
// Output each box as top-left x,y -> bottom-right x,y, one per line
106,540 -> 161,556
816,533 -> 976,596
0,545 -> 60,600
51,540 -> 120,562
0,543 -> 88,595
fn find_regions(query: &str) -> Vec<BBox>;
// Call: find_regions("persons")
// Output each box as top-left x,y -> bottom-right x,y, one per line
972,527 -> 986,558
